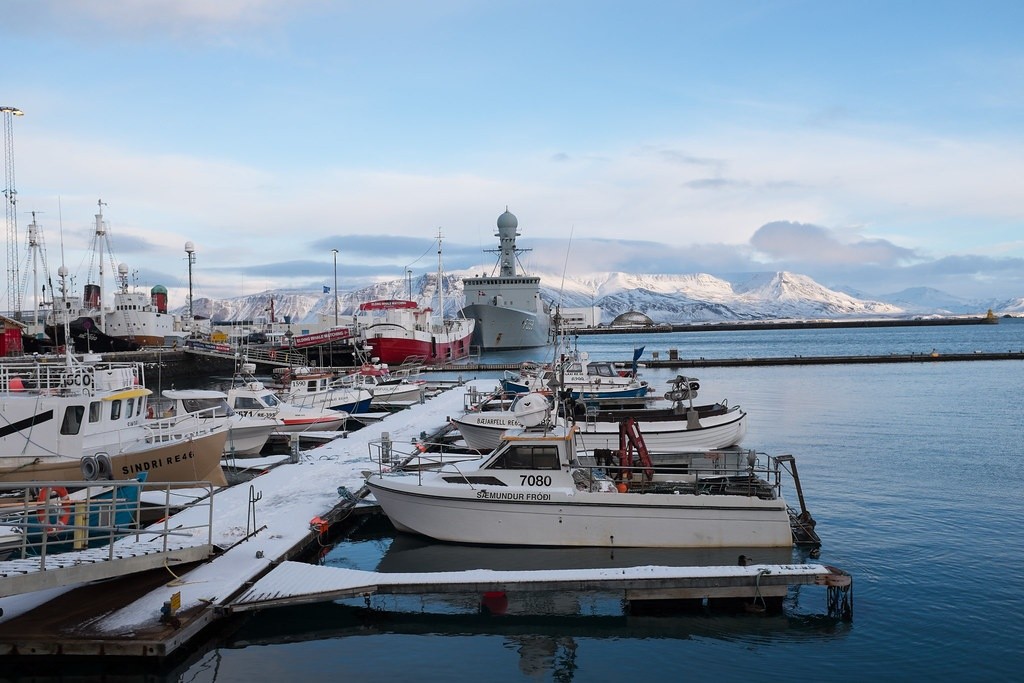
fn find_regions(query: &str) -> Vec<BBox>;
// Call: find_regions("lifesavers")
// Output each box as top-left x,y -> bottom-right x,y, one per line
268,351 -> 276,358
145,404 -> 153,418
38,481 -> 72,533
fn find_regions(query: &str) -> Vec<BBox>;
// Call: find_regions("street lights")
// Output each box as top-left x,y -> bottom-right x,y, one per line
1,105 -> 22,323
185,239 -> 198,319
331,249 -> 339,326
408,270 -> 412,302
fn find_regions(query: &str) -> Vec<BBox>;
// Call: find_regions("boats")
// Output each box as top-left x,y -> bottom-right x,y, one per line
364,392 -> 822,560
457,206 -> 552,352
375,533 -> 792,574
0,195 -> 748,562
